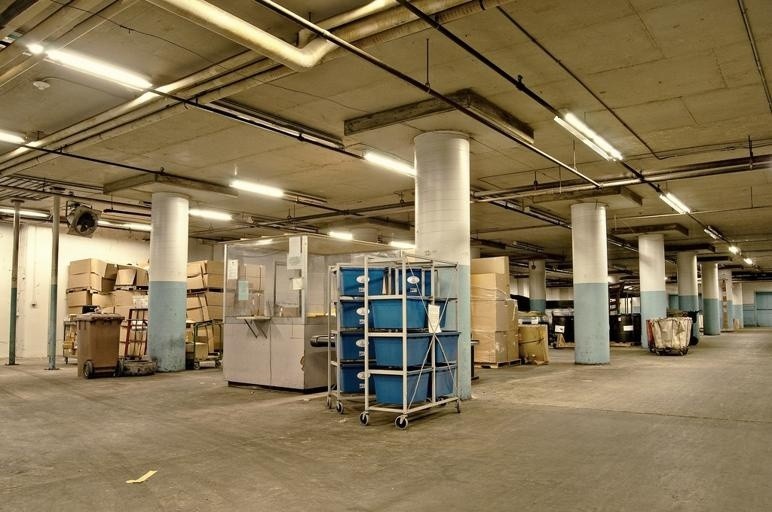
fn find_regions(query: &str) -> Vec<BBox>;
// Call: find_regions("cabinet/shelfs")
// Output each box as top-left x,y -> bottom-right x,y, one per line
326,252 -> 461,429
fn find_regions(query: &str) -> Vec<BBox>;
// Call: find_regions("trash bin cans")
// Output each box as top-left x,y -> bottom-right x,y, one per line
76,314 -> 125,379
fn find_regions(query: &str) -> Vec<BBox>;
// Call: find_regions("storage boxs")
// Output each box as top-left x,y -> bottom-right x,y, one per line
471,273 -> 519,362
337,266 -> 459,405
185,260 -> 264,361
63,259 -> 148,358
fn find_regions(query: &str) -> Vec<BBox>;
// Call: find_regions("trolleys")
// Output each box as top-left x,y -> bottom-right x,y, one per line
185,318 -> 224,371
